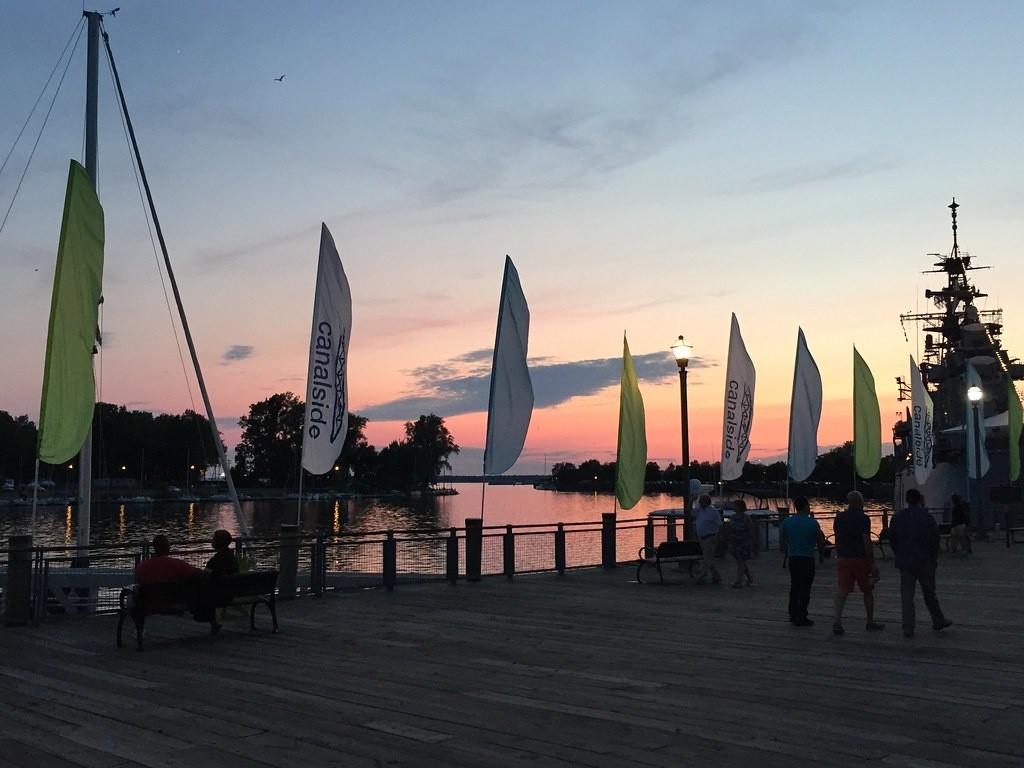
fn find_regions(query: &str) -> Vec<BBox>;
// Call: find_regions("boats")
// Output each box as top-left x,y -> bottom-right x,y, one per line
891,196 -> 1024,507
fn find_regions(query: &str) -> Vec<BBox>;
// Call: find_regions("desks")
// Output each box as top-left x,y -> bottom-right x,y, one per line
637,540 -> 726,585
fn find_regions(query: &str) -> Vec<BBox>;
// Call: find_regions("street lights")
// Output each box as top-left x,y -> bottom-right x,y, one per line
670,334 -> 695,541
968,383 -> 989,541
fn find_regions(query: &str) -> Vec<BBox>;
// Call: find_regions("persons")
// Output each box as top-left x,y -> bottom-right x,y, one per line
724,500 -> 755,587
833,492 -> 884,633
685,495 -> 725,585
206,530 -> 240,631
133,534 -> 195,637
889,490 -> 952,637
780,497 -> 824,627
950,494 -> 969,551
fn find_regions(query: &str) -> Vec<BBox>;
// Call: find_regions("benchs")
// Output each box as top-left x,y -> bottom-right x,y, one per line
115,568 -> 278,653
781,532 -> 890,568
937,521 -> 952,551
1004,511 -> 1024,549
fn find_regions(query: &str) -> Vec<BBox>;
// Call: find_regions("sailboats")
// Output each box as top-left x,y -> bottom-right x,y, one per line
1,6 -> 266,616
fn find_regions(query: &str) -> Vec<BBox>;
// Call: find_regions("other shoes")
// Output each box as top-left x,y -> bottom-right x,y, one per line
866,622 -> 885,631
831,622 -> 845,634
903,626 -> 913,636
711,574 -> 722,584
734,582 -> 744,588
692,580 -> 706,587
789,614 -> 812,626
933,618 -> 952,629
165,608 -> 185,617
944,548 -> 957,552
746,576 -> 754,586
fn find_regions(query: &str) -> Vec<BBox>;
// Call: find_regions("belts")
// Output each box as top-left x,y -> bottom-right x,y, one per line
701,533 -> 716,539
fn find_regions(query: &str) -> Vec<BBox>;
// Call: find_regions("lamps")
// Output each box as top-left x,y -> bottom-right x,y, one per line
690,478 -> 703,498
